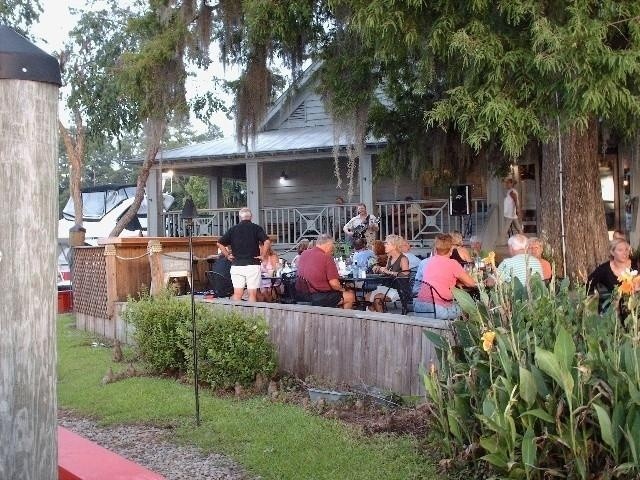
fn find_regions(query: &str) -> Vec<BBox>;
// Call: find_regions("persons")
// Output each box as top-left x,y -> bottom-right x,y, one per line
587,229 -> 640,326
503,179 -> 523,234
209,197 -> 551,320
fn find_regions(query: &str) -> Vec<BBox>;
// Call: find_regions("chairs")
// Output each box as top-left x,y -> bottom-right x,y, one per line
371,270 -> 416,310
408,277 -> 454,319
206,271 -> 233,298
542,277 -> 566,293
283,273 -> 342,307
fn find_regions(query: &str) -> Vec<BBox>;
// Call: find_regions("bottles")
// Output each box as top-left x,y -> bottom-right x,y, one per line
352,261 -> 359,278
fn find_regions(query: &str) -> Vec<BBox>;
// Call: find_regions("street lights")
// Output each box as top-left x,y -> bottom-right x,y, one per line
167,169 -> 174,194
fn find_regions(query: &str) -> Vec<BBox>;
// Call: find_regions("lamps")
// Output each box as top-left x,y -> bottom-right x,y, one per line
279,170 -> 287,185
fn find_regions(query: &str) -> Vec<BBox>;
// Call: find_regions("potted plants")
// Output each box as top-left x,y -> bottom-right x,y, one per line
305,375 -> 355,407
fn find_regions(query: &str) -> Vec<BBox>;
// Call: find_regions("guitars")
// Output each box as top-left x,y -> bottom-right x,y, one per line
353,212 -> 381,239
